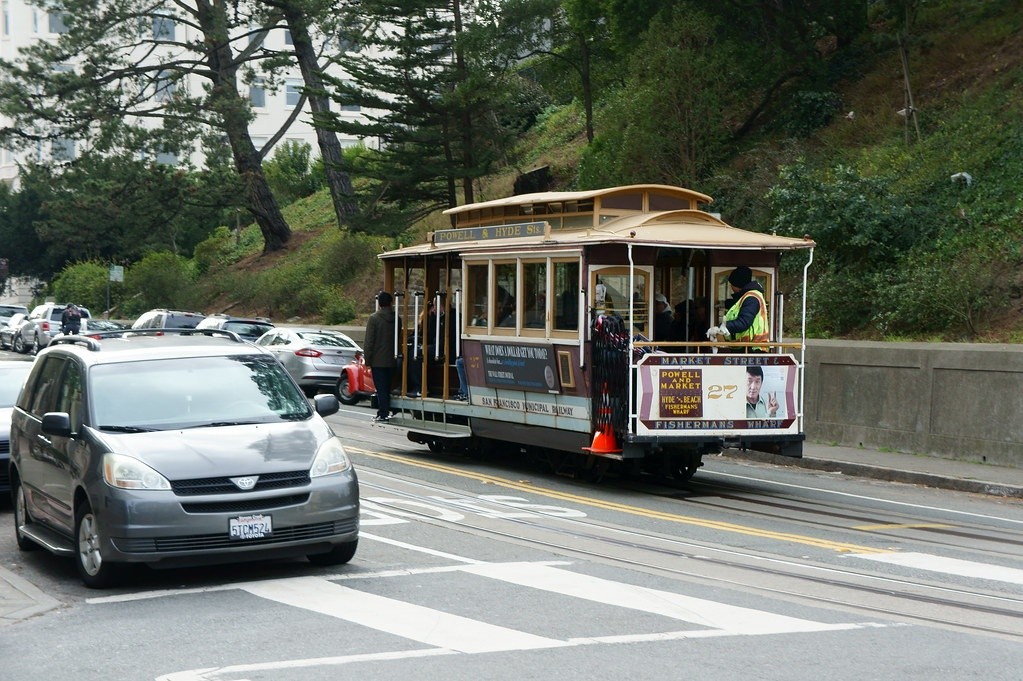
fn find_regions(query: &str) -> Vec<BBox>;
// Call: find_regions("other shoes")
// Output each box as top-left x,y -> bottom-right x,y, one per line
372,415 -> 389,424
453,392 -> 466,401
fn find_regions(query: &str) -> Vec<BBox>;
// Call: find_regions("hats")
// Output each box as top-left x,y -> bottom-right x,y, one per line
656,294 -> 667,303
728,265 -> 752,288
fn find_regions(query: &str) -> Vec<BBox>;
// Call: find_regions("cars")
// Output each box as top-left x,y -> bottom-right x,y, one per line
333,350 -> 379,405
256,326 -> 368,399
0,350 -> 38,500
0,300 -> 128,357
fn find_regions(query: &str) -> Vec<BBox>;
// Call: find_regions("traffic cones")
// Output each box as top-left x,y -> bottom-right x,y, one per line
580,381 -> 624,454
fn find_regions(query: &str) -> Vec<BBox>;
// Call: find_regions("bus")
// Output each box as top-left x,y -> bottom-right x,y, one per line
370,184 -> 816,484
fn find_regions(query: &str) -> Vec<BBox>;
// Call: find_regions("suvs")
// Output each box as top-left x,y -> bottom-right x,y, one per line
7,325 -> 362,591
191,313 -> 275,344
130,307 -> 207,338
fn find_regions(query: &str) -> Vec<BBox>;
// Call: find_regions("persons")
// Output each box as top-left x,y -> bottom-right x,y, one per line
746,366 -> 779,418
62,303 -> 80,344
596,274 -> 706,352
705,267 -> 770,353
480,278 -> 577,330
407,294 -> 456,398
453,300 -> 503,401
364,292 -> 402,423
